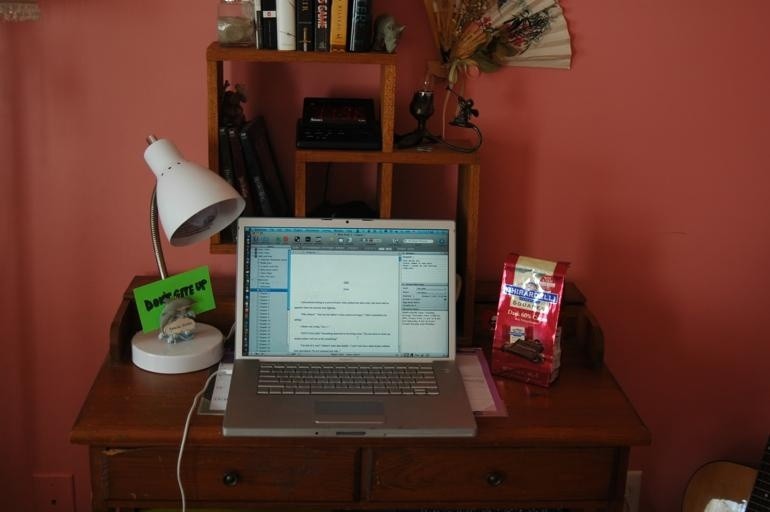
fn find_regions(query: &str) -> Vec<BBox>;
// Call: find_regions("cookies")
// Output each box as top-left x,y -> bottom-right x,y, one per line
509,339 -> 543,359
504,341 -> 545,362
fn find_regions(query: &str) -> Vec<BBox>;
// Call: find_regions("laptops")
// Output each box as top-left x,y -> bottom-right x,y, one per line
222,218 -> 476,437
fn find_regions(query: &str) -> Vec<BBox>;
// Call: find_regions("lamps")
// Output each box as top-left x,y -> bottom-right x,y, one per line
129,133 -> 246,376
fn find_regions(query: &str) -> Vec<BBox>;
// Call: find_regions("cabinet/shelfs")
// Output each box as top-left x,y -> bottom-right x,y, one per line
204,39 -> 482,348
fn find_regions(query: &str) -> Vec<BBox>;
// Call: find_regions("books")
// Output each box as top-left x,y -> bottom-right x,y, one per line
218,113 -> 290,244
253,0 -> 374,54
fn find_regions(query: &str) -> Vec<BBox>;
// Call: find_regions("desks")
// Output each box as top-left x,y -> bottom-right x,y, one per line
68,272 -> 653,512
